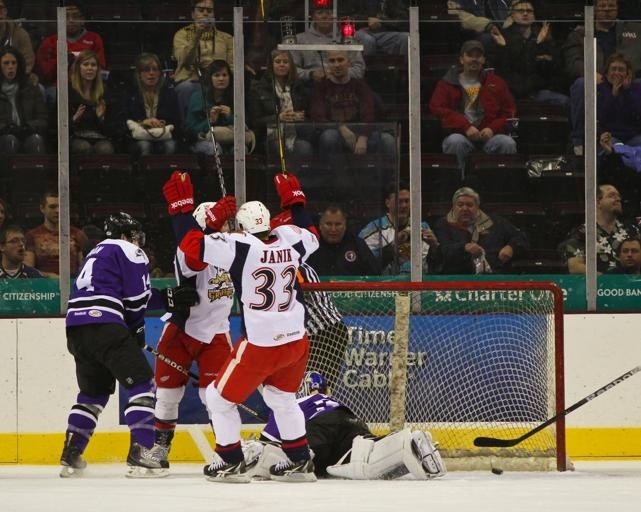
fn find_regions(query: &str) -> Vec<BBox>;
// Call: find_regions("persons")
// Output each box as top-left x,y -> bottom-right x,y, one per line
1,2 -> 641,283
56,175 -> 446,482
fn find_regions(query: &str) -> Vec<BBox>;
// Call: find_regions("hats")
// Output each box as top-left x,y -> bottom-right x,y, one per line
461,40 -> 485,57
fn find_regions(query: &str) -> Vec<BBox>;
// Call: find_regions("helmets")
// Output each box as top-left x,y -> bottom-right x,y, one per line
234,201 -> 272,234
296,370 -> 328,398
104,212 -> 145,248
193,202 -> 217,231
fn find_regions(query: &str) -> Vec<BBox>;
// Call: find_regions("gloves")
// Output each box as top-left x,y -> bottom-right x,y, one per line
162,170 -> 194,215
206,196 -> 236,230
274,173 -> 307,210
162,284 -> 199,314
131,321 -> 146,348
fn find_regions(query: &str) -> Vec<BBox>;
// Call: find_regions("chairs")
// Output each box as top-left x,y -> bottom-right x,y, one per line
511,249 -> 565,270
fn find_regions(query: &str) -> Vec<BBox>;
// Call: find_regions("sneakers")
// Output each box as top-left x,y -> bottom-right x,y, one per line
59,446 -> 87,468
411,430 -> 440,474
127,442 -> 169,469
204,458 -> 246,478
270,451 -> 315,476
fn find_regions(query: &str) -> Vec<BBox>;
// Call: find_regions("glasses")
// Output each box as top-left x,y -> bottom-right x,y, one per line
5,238 -> 27,245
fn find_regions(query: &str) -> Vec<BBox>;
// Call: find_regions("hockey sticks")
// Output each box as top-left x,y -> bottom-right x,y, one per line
475,367 -> 640,447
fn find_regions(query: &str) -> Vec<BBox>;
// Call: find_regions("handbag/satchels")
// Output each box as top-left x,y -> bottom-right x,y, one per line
127,119 -> 174,141
